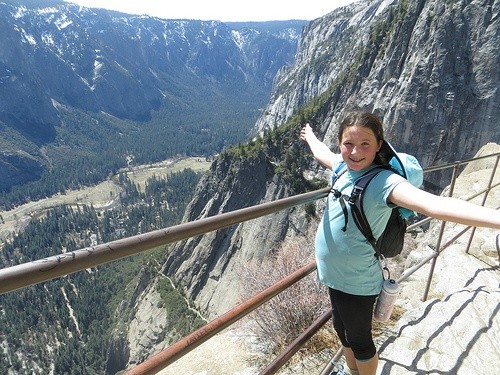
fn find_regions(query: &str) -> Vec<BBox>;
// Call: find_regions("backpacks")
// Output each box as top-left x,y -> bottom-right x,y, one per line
330,159 -> 407,260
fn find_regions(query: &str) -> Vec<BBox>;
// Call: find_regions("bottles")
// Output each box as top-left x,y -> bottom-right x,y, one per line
374,280 -> 400,323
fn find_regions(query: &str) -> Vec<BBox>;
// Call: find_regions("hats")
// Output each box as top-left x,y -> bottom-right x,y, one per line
377,139 -> 424,219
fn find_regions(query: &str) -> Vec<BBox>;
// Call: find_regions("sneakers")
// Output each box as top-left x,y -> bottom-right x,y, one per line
328,362 -> 358,375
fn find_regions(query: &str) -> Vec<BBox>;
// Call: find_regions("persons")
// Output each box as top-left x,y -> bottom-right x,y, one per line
300,111 -> 500,375
